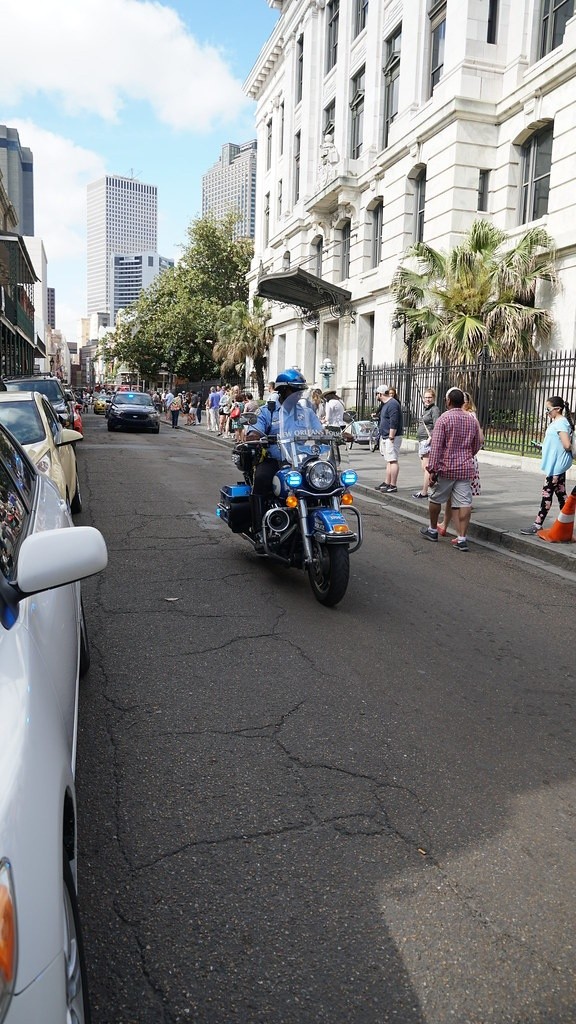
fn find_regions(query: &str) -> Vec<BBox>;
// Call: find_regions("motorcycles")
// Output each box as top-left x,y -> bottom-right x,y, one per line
215,386 -> 360,609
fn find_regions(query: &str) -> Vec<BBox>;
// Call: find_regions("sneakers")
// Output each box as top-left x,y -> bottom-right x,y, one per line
419,526 -> 438,541
520,524 -> 543,535
411,493 -> 427,499
453,541 -> 468,550
375,482 -> 389,490
381,484 -> 397,493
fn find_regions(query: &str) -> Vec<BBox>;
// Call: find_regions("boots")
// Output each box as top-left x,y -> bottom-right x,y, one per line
249,492 -> 267,554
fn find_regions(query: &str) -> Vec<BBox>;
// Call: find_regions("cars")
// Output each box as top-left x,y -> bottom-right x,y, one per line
0,423 -> 110,1024
64,388 -> 82,434
0,390 -> 82,512
93,396 -> 112,415
105,392 -> 161,434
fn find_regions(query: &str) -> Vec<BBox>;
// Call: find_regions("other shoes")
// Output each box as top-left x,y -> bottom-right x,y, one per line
437,525 -> 446,535
451,538 -> 458,544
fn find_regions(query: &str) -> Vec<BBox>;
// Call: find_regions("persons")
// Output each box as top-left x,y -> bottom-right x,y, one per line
245,368 -> 354,552
519,396 -> 576,534
266,382 -> 279,404
418,387 -> 487,551
311,389 -> 346,434
411,389 -> 440,501
74,384 -> 203,429
0,488 -> 25,579
373,385 -> 403,493
370,388 -> 401,447
205,383 -> 259,445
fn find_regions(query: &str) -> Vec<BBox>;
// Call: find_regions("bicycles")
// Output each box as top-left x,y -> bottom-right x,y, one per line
369,416 -> 383,452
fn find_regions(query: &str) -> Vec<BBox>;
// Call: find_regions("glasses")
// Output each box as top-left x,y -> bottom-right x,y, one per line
545,407 -> 561,411
287,388 -> 303,393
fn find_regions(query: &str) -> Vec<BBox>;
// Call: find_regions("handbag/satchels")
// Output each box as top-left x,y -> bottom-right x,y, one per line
230,403 -> 241,419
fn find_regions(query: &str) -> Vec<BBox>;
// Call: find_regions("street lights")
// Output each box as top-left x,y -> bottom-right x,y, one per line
395,311 -> 416,427
319,357 -> 337,389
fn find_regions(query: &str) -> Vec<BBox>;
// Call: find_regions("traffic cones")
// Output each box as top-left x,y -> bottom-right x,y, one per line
536,486 -> 575,544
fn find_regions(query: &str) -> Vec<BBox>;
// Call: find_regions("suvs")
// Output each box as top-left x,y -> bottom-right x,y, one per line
3,372 -> 76,435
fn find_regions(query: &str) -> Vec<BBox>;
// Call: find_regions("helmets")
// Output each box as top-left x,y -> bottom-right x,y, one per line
274,369 -> 309,389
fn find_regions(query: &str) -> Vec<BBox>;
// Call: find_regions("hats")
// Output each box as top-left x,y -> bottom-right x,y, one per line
373,384 -> 389,396
319,388 -> 336,398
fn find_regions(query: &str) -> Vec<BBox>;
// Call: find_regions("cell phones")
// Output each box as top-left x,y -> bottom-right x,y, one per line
531,440 -> 543,449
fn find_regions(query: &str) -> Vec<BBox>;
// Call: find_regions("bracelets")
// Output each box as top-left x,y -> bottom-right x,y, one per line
389,437 -> 394,440
